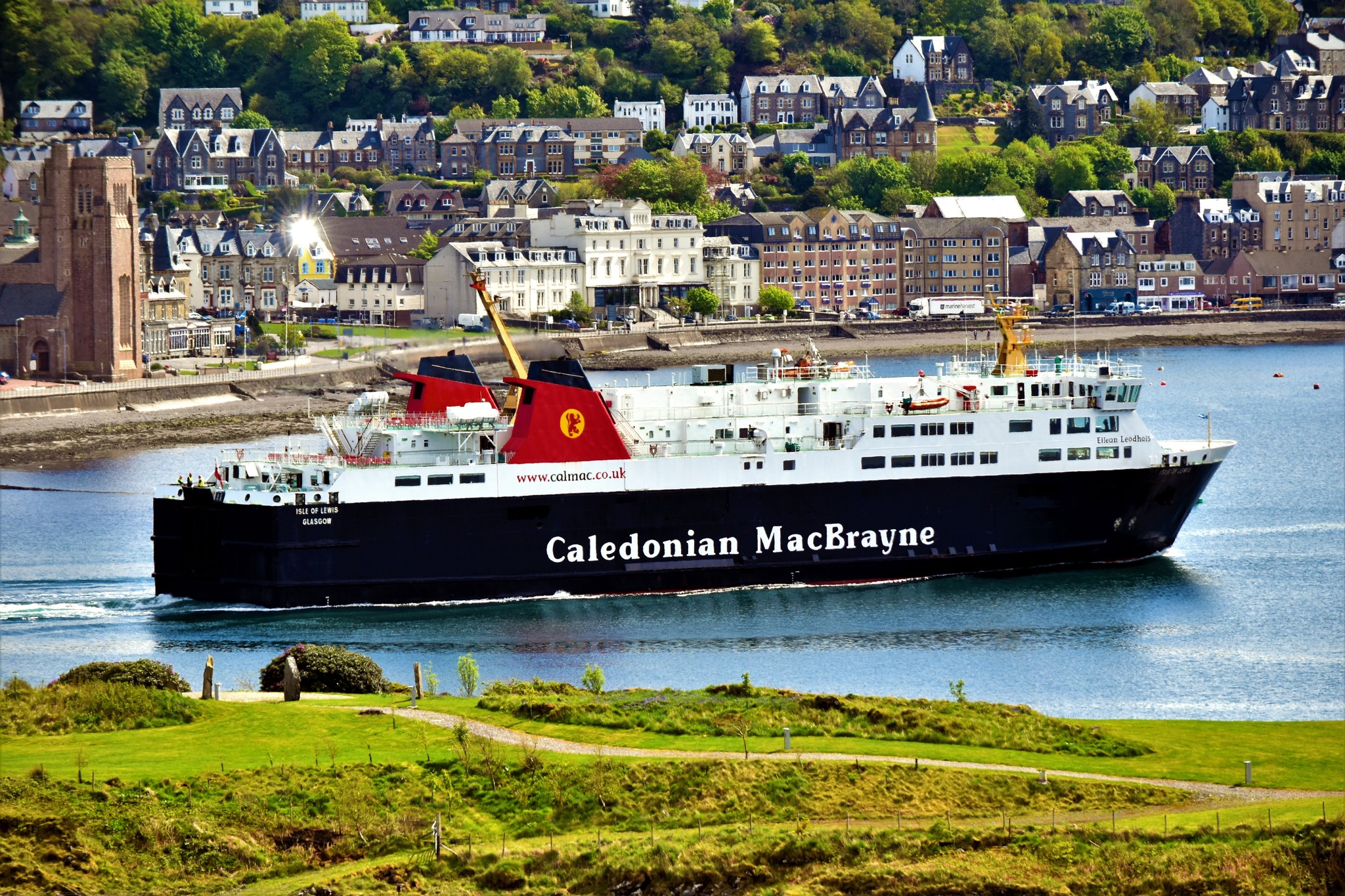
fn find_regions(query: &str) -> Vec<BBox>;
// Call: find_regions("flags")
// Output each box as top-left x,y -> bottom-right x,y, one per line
239,311 -> 246,319
994,308 -> 1005,314
1198,414 -> 1209,420
281,306 -> 287,311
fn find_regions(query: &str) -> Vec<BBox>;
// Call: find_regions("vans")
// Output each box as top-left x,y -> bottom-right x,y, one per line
457,314 -> 485,329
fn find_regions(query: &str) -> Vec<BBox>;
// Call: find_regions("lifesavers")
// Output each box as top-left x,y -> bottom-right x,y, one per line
885,403 -> 893,412
235,450 -> 244,460
650,445 -> 657,455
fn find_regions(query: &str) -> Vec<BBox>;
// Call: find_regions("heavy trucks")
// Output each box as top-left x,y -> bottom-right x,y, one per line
909,296 -> 985,321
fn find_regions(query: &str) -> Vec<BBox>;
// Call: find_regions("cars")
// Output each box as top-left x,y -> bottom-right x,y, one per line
1043,298 -> 1283,319
725,315 -> 739,322
681,315 -> 694,324
892,307 -> 909,316
143,354 -> 152,363
562,320 -> 580,329
763,314 -> 774,320
604,314 -> 638,324
819,308 -> 881,320
1331,300 -> 1345,308
0,371 -> 10,385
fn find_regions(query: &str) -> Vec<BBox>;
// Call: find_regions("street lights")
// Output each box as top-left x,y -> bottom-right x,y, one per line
15,317 -> 24,378
375,295 -> 386,336
286,294 -> 298,322
361,284 -> 365,319
47,329 -> 67,385
440,288 -> 448,327
414,291 -> 422,326
444,280 -> 455,330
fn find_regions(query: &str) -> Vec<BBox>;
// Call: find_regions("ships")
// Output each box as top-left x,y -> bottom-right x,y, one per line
151,268 -> 1240,608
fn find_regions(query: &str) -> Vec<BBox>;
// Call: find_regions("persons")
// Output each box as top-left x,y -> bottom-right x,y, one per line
623,322 -> 631,334
974,330 -> 977,340
462,335 -> 467,345
238,364 -> 243,372
195,364 -> 201,375
219,361 -> 229,367
534,327 -> 538,335
1264,298 -> 1295,310
271,313 -> 300,321
229,357 -> 234,363
843,312 -> 876,324
454,318 -> 458,329
960,310 -> 965,321
987,329 -> 990,340
276,347 -> 295,358
694,315 -> 751,330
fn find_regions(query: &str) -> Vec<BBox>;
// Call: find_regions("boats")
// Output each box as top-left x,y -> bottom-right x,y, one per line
770,358 -> 814,377
829,360 -> 855,373
897,398 -> 950,410
